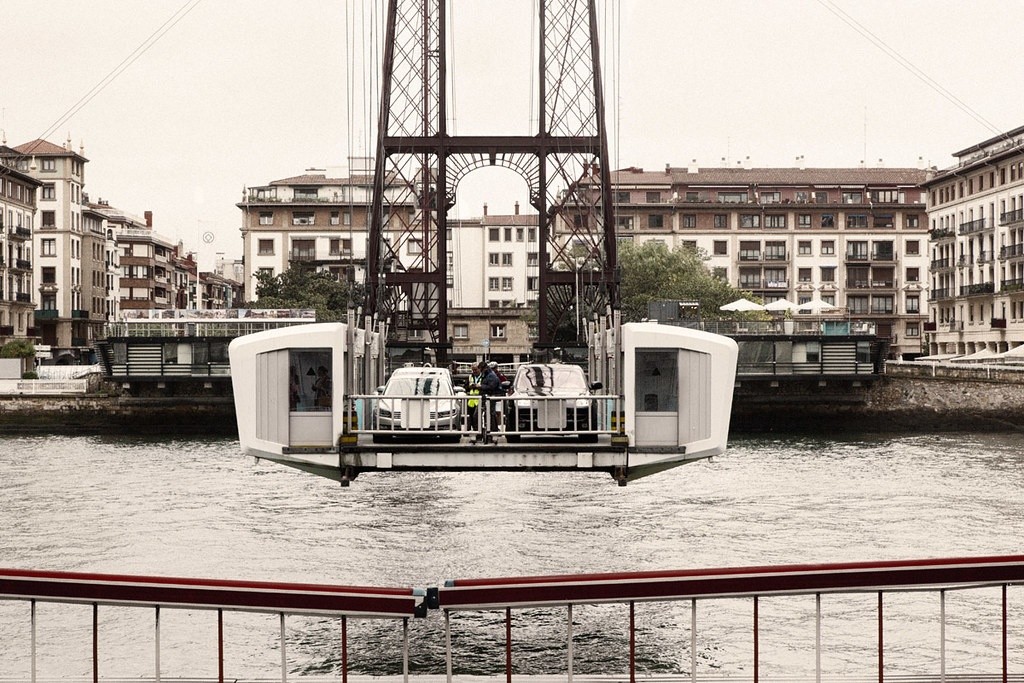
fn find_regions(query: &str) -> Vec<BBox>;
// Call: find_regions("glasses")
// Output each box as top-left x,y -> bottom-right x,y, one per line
480,368 -> 484,372
490,366 -> 497,370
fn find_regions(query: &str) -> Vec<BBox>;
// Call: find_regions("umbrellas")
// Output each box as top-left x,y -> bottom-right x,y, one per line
762,298 -> 800,310
718,297 -> 766,311
801,298 -> 841,311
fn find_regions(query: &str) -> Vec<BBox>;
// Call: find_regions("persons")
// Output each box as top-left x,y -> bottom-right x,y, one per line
463,362 -> 509,444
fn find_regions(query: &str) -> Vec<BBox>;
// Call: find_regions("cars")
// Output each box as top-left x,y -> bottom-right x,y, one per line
372,366 -> 467,443
507,362 -> 603,443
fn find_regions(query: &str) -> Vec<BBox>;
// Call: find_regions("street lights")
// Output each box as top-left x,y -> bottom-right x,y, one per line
191,251 -> 199,337
